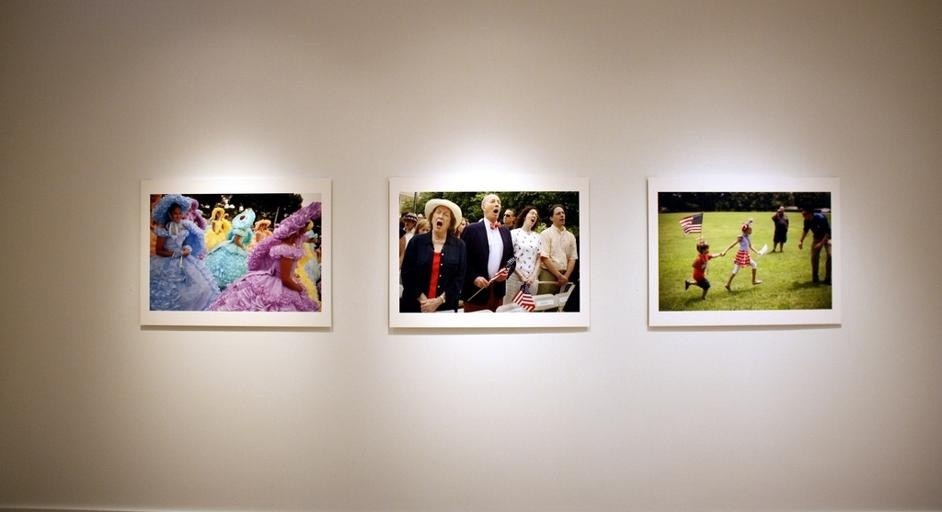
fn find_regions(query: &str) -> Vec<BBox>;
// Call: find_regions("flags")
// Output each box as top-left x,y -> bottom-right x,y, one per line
680,213 -> 703,235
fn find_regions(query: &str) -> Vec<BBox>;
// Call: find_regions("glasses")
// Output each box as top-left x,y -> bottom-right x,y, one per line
503,214 -> 510,217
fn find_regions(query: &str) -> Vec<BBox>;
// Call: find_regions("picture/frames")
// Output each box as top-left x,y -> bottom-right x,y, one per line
387,175 -> 591,329
645,176 -> 844,329
138,177 -> 334,327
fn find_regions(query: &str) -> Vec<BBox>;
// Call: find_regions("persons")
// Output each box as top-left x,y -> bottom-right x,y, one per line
799,205 -> 831,286
685,240 -> 723,299
722,224 -> 763,290
771,206 -> 788,253
399,191 -> 578,313
150,194 -> 321,311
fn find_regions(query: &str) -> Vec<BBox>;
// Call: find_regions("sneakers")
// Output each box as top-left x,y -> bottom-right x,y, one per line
685,279 -> 690,289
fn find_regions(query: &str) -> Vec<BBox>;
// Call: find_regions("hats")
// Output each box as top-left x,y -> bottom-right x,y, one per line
246,201 -> 321,271
231,208 -> 255,230
403,212 -> 418,223
424,198 -> 462,229
211,208 -> 224,221
253,219 -> 270,230
152,194 -> 206,230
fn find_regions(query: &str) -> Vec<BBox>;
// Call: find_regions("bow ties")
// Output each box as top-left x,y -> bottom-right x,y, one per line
489,222 -> 501,229
